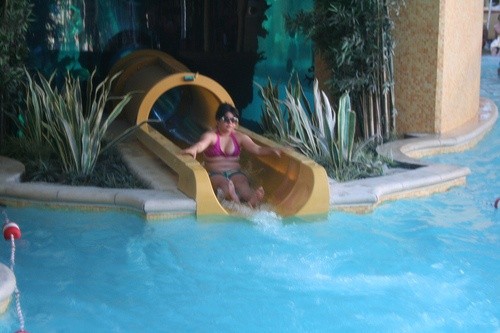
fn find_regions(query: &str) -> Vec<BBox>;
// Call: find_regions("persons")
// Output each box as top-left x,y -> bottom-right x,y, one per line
181,102 -> 292,208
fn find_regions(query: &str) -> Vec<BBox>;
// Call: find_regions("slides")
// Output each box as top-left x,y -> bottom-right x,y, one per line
107,49 -> 329,222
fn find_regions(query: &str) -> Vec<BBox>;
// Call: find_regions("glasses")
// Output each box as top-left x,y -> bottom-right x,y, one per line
219,116 -> 238,123
